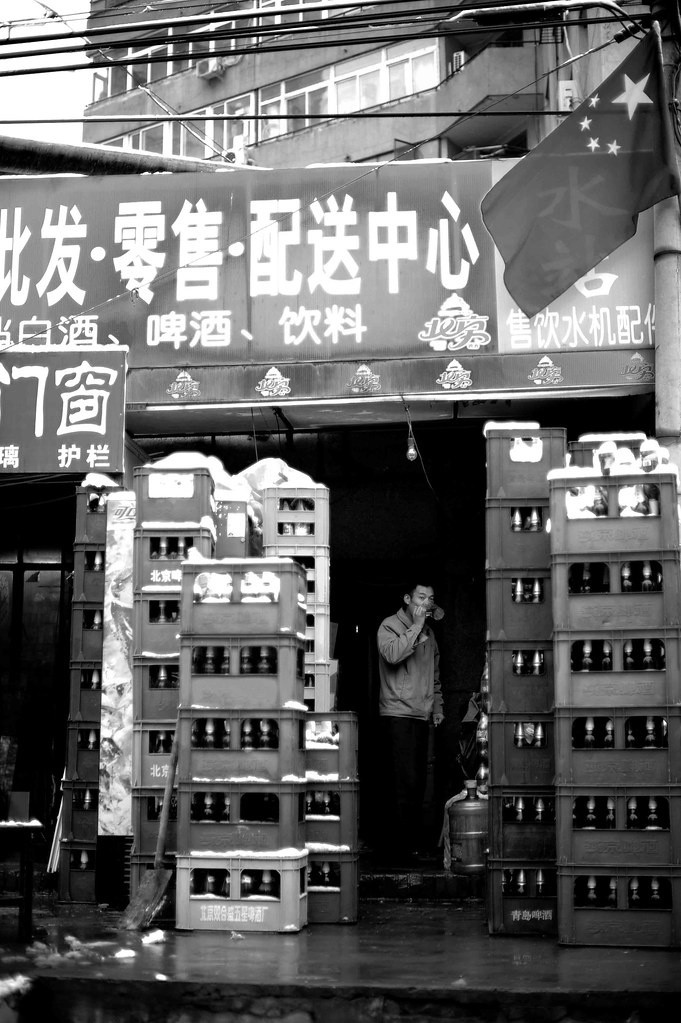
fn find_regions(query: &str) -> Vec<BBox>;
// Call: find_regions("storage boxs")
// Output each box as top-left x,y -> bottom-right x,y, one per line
484,429 -> 681,947
64,467 -> 360,934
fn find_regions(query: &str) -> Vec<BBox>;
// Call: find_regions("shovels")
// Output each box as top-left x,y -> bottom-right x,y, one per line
111,704 -> 180,933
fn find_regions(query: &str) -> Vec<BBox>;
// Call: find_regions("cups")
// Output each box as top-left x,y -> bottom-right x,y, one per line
421,601 -> 444,620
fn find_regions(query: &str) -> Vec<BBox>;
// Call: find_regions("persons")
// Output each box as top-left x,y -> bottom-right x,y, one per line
376,580 -> 446,871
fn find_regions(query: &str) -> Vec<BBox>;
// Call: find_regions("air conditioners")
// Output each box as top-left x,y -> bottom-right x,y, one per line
221,147 -> 249,165
453,51 -> 471,74
555,80 -> 573,120
195,57 -> 225,79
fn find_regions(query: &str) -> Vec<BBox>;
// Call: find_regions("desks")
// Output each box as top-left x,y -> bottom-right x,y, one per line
0,820 -> 50,942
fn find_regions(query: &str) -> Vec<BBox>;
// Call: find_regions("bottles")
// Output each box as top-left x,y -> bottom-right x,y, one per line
73,495 -> 333,896
447,778 -> 487,871
475,436 -> 669,912
